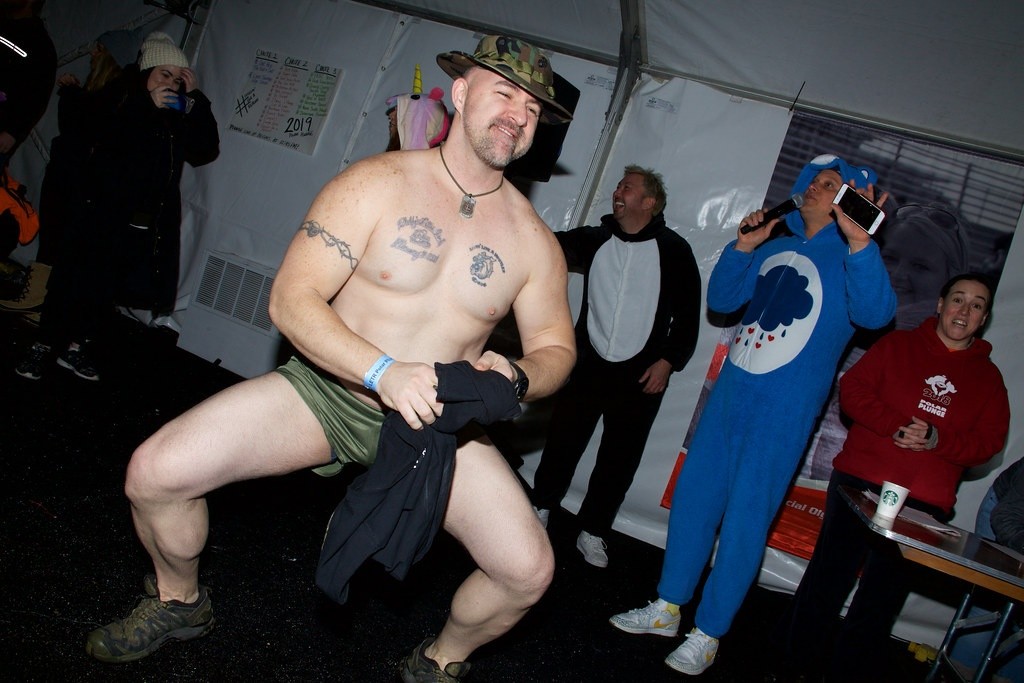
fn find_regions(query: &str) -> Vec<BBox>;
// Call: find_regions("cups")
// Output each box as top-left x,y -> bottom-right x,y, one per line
876,481 -> 911,519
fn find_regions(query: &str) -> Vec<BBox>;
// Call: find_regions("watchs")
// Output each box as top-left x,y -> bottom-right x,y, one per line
510,361 -> 529,400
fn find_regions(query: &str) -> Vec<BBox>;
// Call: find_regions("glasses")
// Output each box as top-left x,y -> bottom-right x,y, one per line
893,203 -> 960,245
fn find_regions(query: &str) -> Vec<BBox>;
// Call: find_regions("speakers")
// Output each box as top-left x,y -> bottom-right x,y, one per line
502,71 -> 582,183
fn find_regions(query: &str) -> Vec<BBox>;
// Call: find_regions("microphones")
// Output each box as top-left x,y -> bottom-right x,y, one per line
740,191 -> 805,235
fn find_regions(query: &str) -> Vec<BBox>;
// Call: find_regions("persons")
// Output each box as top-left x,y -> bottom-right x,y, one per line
15,29 -> 220,413
86,35 -> 577,683
609,153 -> 898,675
532,165 -> 702,567
949,456 -> 1024,683
763,273 -> 1010,683
879,202 -> 1016,331
384,64 -> 449,152
0,0 -> 58,159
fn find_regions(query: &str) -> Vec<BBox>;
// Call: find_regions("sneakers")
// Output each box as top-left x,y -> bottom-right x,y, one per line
576,529 -> 609,568
398,637 -> 473,683
531,504 -> 550,529
15,341 -> 49,380
608,599 -> 680,638
663,623 -> 720,676
57,347 -> 100,381
84,576 -> 217,663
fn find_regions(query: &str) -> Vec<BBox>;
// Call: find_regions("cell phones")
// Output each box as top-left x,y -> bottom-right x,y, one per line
834,184 -> 885,234
164,89 -> 195,113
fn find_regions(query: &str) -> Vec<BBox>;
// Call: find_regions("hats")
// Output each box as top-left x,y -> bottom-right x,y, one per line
138,30 -> 190,69
436,34 -> 574,127
94,28 -> 140,72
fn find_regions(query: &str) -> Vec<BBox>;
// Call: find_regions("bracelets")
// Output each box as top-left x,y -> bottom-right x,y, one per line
364,354 -> 396,391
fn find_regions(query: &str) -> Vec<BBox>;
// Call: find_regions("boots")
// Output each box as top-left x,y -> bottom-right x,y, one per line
15,311 -> 44,329
0,259 -> 53,314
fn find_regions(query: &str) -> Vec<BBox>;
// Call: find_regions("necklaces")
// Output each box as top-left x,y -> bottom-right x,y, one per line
440,145 -> 504,218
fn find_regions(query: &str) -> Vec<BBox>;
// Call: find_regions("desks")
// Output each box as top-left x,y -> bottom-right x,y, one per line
833,482 -> 1024,683
